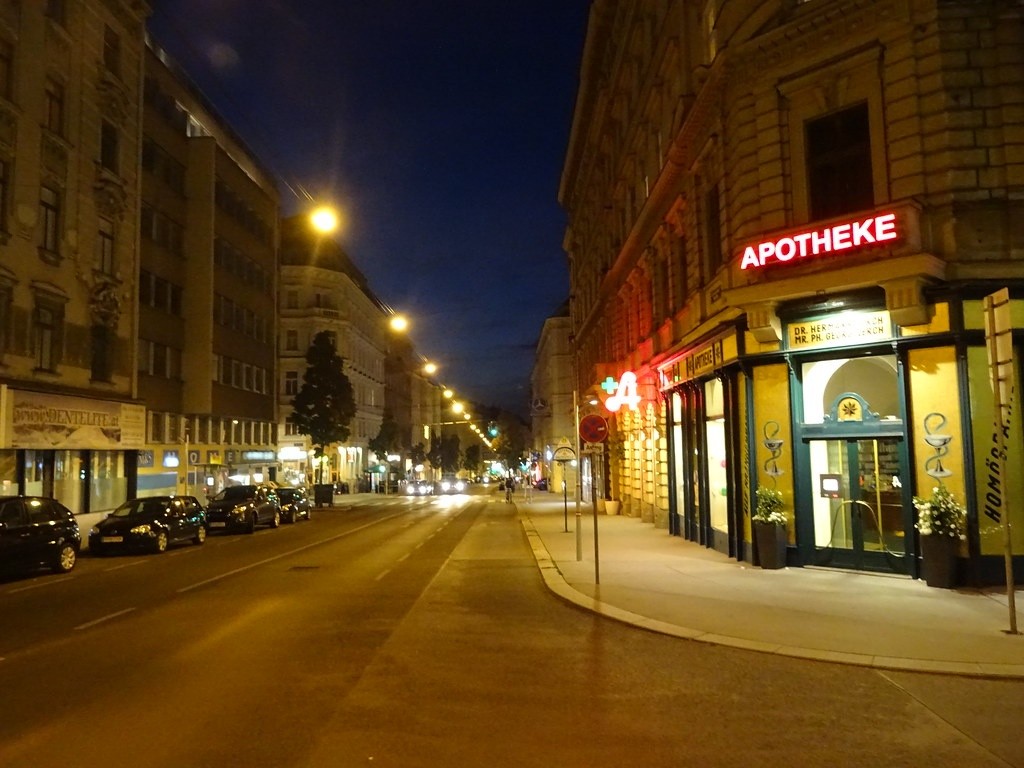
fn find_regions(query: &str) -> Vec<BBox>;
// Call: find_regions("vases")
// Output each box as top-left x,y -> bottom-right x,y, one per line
605,500 -> 620,516
920,533 -> 962,589
596,496 -> 608,512
755,524 -> 787,569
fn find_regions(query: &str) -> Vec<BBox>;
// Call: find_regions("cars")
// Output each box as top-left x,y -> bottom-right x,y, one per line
202,485 -> 281,534
0,496 -> 82,581
89,495 -> 207,552
275,487 -> 312,523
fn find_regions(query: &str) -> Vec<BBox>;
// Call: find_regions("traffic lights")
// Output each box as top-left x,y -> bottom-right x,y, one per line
489,422 -> 498,438
521,458 -> 527,472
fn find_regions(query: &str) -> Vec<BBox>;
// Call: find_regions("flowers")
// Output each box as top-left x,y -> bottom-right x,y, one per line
912,485 -> 968,541
752,486 -> 787,527
